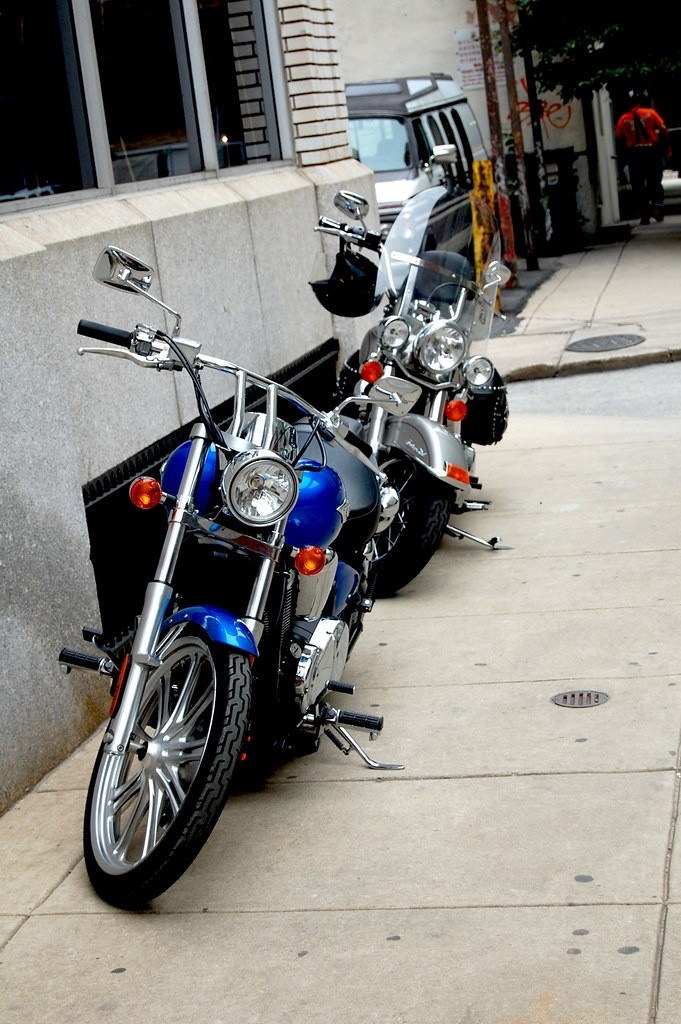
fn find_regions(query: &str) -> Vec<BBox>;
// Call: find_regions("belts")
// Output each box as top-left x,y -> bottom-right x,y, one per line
635,143 -> 653,147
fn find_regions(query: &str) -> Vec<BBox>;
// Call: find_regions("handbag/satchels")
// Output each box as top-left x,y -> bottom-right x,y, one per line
461,366 -> 509,446
332,348 -> 366,420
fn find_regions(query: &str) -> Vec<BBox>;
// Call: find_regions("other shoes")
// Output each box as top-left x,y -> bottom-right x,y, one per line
654,209 -> 663,223
640,212 -> 650,225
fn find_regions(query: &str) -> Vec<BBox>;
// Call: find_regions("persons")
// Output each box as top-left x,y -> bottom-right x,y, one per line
615,97 -> 666,225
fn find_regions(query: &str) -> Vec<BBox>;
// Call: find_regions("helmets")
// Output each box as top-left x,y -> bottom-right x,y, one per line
308,252 -> 384,317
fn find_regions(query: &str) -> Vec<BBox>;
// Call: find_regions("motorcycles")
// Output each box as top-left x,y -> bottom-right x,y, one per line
315,187 -> 512,601
55,245 -> 424,911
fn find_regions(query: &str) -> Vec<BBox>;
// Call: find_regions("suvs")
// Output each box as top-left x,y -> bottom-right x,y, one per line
345,74 -> 495,318
